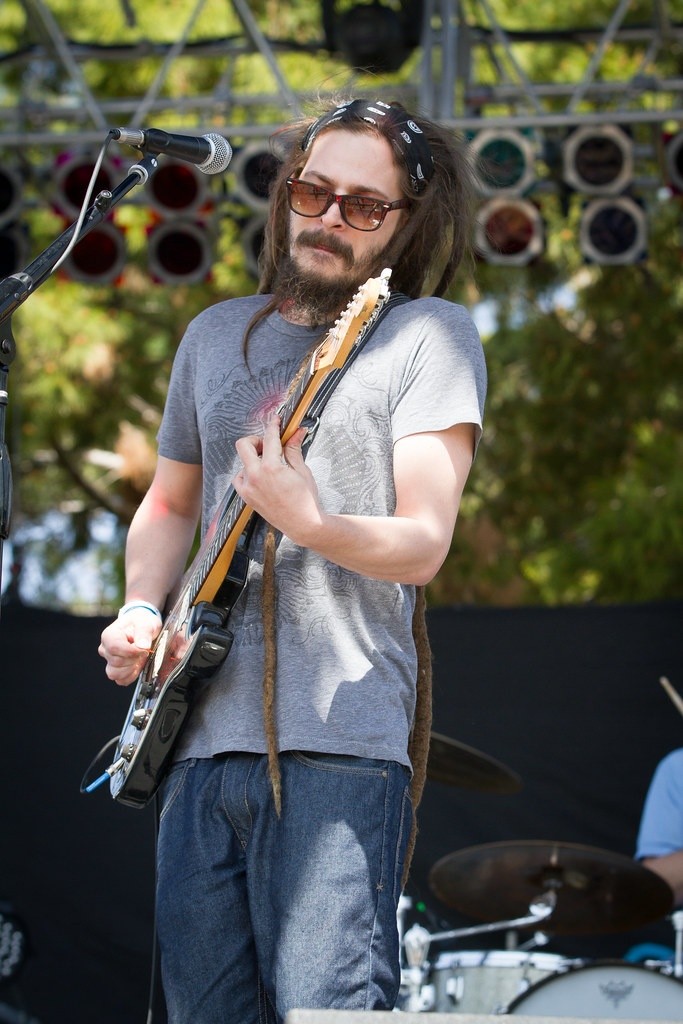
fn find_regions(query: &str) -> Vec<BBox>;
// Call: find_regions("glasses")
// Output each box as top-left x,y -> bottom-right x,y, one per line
286,168 -> 414,232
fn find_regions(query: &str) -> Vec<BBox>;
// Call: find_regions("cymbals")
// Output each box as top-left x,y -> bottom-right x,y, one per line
427,731 -> 523,796
427,840 -> 671,932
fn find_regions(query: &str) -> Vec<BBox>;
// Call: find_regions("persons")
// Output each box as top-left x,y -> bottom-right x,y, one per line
627,741 -> 683,980
95,97 -> 489,1023
116,601 -> 163,628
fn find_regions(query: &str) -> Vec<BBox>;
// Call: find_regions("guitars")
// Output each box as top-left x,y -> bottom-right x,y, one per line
108,265 -> 393,810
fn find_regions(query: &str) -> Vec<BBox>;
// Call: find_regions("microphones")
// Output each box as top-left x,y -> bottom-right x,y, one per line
113,127 -> 234,175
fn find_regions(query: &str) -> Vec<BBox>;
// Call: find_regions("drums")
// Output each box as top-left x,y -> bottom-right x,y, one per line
402,951 -> 683,1024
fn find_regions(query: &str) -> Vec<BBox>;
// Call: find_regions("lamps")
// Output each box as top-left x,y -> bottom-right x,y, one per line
139,150 -> 214,218
459,130 -> 535,196
665,131 -> 682,195
55,152 -> 118,222
146,211 -> 210,288
64,214 -> 126,284
575,194 -> 650,265
558,123 -> 635,192
319,1 -> 427,76
244,213 -> 275,274
0,223 -> 27,284
234,139 -> 293,211
0,162 -> 31,228
473,187 -> 545,266
